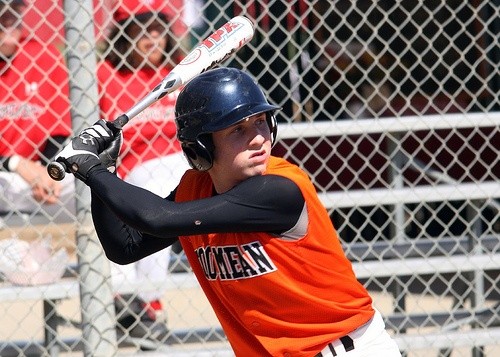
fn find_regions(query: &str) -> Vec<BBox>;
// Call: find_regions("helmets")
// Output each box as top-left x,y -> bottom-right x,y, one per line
175,67 -> 283,172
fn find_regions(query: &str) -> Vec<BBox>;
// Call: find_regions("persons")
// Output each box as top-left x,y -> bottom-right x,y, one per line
65,67 -> 403,356
90,1 -> 191,348
0,1 -> 63,205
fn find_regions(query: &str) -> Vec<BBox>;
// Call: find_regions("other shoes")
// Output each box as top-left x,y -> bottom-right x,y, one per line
141,299 -> 166,324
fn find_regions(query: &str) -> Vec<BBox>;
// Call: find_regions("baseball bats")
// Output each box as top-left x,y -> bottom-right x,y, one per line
46,15 -> 255,181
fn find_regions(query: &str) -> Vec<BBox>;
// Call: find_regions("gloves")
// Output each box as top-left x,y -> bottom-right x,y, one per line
56,135 -> 103,182
86,118 -> 122,179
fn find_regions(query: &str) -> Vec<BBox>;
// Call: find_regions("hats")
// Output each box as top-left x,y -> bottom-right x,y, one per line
113,0 -> 170,22
